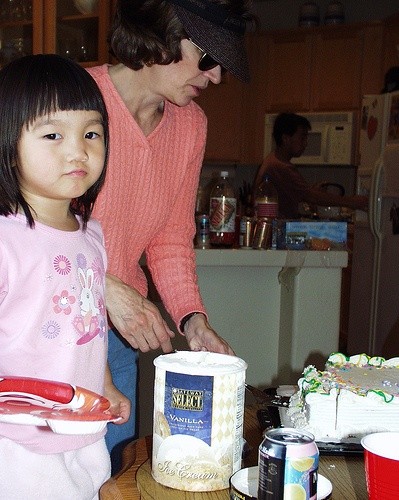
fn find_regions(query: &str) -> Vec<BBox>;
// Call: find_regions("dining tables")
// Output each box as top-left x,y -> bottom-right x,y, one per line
99,401 -> 370,500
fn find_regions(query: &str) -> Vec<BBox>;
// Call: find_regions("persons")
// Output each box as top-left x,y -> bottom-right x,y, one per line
75,0 -> 253,471
248,109 -> 368,216
0,55 -> 134,500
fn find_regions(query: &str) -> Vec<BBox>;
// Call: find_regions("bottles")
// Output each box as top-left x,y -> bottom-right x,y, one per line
252,173 -> 280,248
208,170 -> 241,248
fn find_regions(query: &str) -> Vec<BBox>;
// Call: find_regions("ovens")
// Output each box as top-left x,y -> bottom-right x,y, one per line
263,109 -> 354,167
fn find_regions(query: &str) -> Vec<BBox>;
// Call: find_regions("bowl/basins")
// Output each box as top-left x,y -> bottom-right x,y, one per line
228,464 -> 334,500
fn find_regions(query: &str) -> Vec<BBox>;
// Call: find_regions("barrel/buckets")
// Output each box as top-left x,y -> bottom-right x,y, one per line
151,350 -> 247,493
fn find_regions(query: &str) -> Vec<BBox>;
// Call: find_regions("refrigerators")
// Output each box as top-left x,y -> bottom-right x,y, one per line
346,88 -> 399,362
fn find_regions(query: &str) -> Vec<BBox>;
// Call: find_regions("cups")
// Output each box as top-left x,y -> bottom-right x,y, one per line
0,0 -> 97,68
359,431 -> 399,500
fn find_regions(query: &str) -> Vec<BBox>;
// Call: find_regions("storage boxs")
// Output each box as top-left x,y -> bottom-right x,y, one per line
273,219 -> 348,250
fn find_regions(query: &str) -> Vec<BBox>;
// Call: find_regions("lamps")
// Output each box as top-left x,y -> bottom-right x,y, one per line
299,3 -> 345,31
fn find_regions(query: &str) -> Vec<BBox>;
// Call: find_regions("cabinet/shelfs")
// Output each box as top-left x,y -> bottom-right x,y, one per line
193,11 -> 399,169
0,0 -> 116,67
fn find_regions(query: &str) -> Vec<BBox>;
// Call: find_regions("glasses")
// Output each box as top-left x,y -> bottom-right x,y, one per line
188,38 -> 227,75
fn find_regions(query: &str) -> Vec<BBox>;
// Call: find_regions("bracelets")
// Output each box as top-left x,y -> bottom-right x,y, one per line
180,312 -> 197,332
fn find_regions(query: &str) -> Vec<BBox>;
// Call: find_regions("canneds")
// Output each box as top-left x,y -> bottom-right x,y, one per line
194,213 -> 210,246
256,427 -> 318,500
239,216 -> 271,251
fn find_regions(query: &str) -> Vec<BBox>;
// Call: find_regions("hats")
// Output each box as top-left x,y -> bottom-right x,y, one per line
162,1 -> 250,83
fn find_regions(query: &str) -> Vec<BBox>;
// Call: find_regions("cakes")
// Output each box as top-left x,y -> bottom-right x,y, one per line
287,351 -> 399,441
